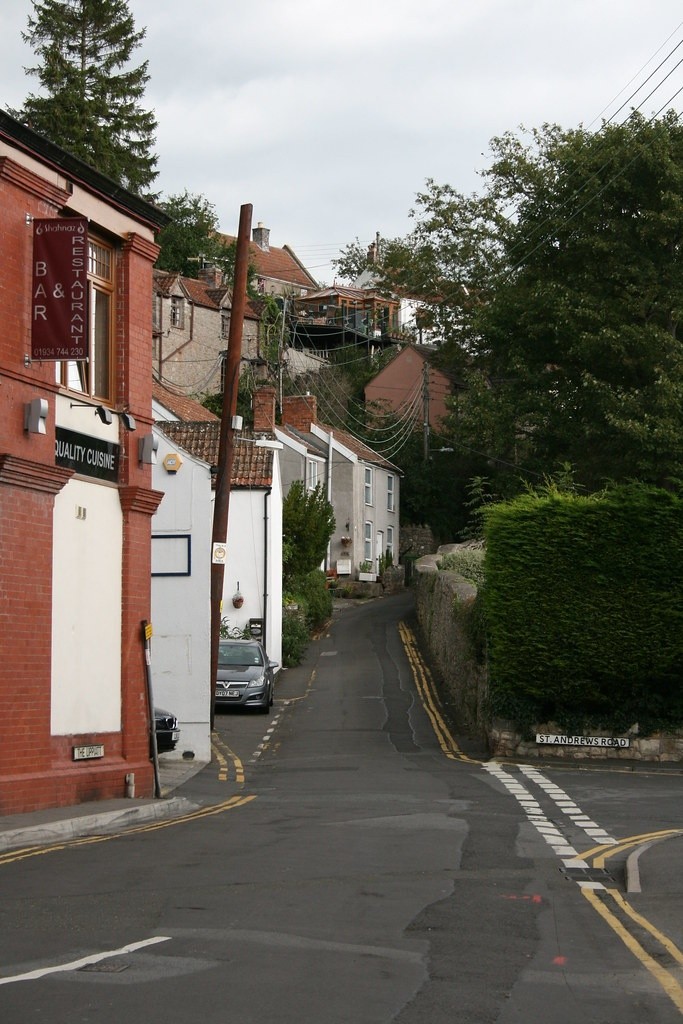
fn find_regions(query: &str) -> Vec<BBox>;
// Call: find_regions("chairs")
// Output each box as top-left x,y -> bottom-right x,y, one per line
243,652 -> 255,662
218,652 -> 225,663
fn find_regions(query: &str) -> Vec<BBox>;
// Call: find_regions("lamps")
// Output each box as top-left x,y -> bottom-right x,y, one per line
70,403 -> 113,425
94,411 -> 137,432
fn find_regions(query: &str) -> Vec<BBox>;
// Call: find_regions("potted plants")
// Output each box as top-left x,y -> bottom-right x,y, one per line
359,561 -> 377,582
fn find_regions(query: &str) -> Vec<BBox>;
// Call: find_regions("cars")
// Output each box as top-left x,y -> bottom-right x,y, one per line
148,705 -> 180,758
215,639 -> 278,714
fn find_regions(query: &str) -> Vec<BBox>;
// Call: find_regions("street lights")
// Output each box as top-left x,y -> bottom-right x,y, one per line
211,356 -> 267,728
